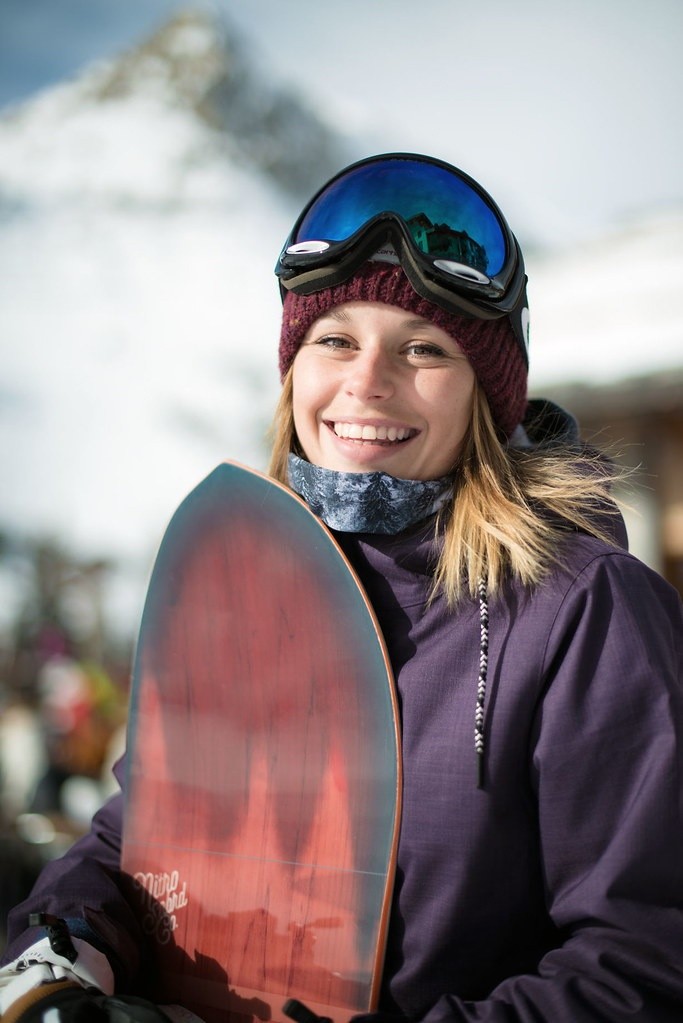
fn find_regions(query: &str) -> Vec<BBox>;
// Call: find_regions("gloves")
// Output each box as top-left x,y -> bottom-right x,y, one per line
0,912 -> 116,1022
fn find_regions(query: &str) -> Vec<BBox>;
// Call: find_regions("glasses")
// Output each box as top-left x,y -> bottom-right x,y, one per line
275,151 -> 530,370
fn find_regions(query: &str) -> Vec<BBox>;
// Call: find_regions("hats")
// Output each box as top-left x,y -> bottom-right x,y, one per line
278,243 -> 530,437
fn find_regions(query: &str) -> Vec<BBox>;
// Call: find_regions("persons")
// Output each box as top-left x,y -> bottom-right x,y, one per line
2,152 -> 681,1023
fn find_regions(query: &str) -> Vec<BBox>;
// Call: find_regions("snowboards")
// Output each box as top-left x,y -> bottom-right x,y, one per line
119,461 -> 404,1023
